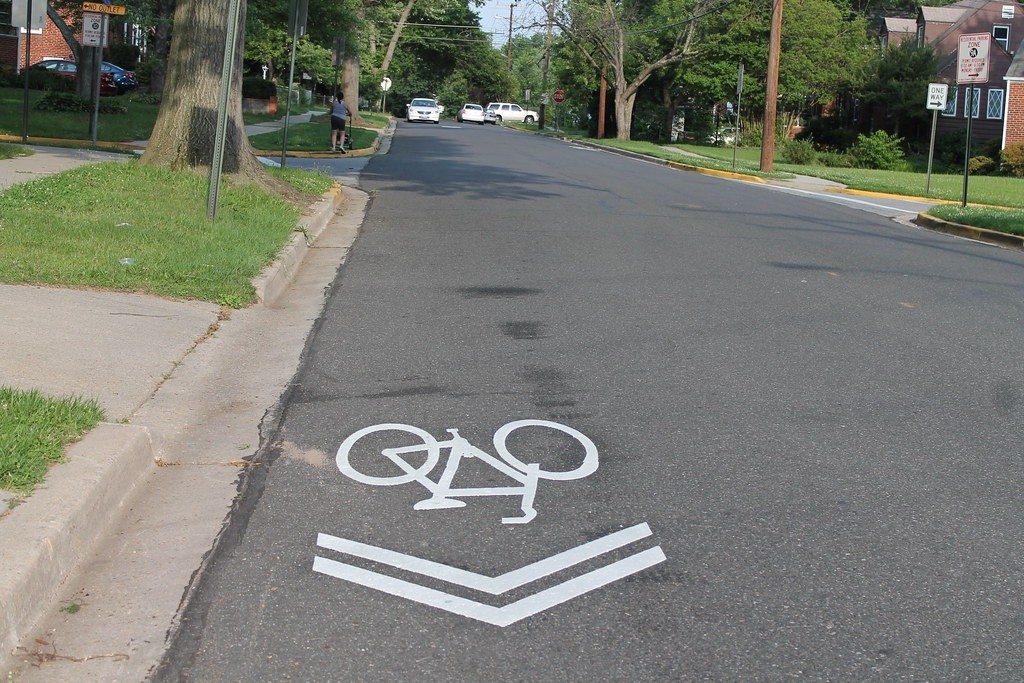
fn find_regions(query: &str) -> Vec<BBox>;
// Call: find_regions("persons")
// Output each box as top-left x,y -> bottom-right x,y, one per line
328,92 -> 352,154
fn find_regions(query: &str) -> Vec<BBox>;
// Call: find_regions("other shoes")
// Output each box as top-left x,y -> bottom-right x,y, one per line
339,146 -> 346,154
329,147 -> 335,151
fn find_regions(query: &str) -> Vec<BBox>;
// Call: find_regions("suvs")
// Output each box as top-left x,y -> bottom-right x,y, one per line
486,102 -> 539,124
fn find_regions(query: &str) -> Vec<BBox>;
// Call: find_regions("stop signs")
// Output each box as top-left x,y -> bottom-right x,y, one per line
553,90 -> 565,103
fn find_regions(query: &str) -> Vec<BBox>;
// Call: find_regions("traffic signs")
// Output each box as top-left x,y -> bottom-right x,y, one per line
926,83 -> 948,110
82,1 -> 126,15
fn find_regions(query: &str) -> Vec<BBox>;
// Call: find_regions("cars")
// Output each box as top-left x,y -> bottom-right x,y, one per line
457,104 -> 484,125
20,60 -> 118,97
483,108 -> 496,125
101,61 -> 138,91
406,98 -> 441,124
